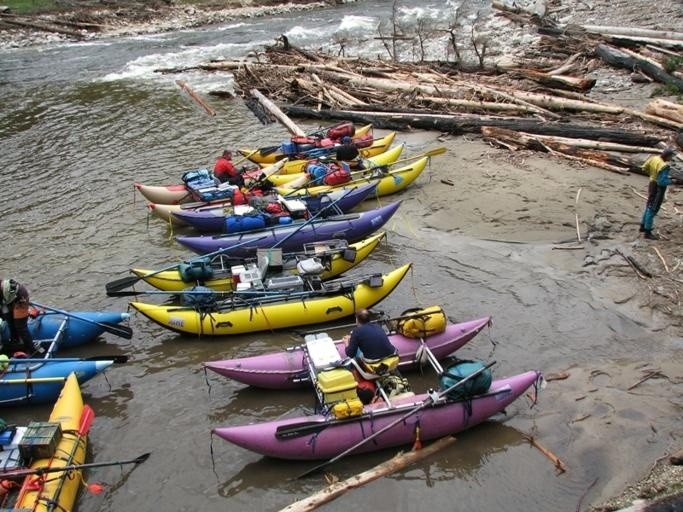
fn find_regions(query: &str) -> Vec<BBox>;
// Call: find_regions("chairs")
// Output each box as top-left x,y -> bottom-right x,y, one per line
348,357 -> 391,382
275,192 -> 308,214
237,254 -> 270,285
209,173 -> 229,189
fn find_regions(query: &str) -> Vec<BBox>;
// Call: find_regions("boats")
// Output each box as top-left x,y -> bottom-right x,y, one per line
194,304 -> 541,461
1,305 -> 132,407
169,178 -> 402,256
263,142 -> 431,202
128,230 -> 412,336
1,372 -> 94,512
235,122 -> 397,175
131,156 -> 314,230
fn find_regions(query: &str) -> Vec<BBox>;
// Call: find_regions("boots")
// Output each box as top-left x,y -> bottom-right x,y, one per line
640,224 -> 658,239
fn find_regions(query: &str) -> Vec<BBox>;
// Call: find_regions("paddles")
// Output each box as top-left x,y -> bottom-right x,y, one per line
105,236 -> 266,292
107,289 -> 357,311
49,404 -> 96,511
0,452 -> 150,478
181,198 -> 232,210
276,137 -> 385,162
260,121 -> 345,156
351,147 -> 445,175
29,301 -> 132,340
276,360 -> 511,478
0,355 -> 127,363
289,310 -> 441,337
213,213 -> 360,239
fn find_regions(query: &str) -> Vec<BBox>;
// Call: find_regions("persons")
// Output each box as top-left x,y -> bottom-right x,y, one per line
335,136 -> 362,171
0,277 -> 37,357
342,306 -> 401,379
636,148 -> 675,242
211,148 -> 245,190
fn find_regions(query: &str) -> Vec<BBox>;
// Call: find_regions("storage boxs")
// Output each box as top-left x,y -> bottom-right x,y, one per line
256,246 -> 283,275
313,370 -> 360,406
15,418 -> 63,458
